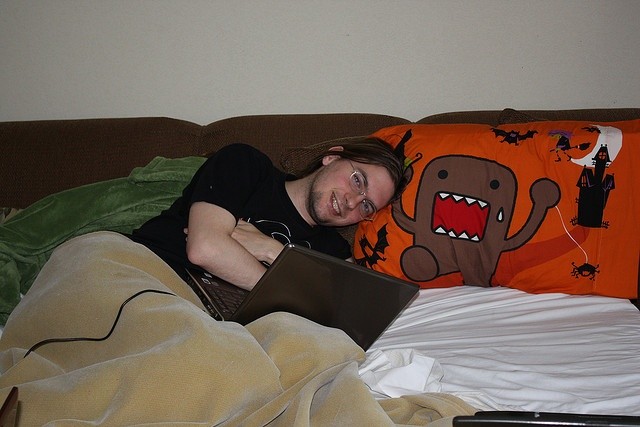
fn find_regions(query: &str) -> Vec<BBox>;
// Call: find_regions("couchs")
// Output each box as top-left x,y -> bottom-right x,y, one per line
1,106 -> 636,426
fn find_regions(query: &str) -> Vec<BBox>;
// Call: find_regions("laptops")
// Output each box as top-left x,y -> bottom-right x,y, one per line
183,243 -> 420,352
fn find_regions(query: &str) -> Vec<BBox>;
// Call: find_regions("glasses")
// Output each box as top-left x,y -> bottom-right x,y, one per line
348,156 -> 377,222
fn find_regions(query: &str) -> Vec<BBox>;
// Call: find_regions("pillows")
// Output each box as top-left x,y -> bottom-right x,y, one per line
351,122 -> 635,299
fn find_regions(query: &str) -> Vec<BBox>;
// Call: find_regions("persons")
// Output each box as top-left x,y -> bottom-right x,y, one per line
127,134 -> 405,292
